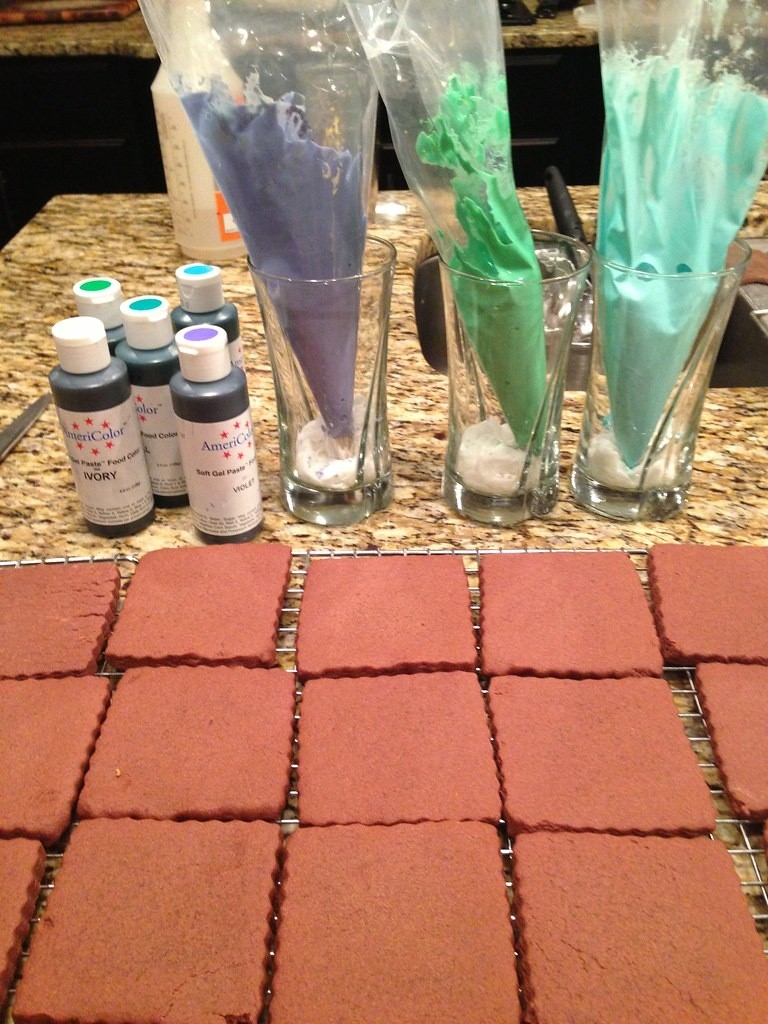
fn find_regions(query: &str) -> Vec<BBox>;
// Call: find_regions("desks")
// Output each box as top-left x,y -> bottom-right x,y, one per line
0,183 -> 768,1024
0,0 -> 768,232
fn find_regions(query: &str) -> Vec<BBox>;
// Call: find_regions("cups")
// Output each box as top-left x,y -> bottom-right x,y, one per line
247,235 -> 397,526
571,236 -> 751,522
438,228 -> 593,527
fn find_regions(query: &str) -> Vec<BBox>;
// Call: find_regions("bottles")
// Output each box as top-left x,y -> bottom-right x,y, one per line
149,0 -> 249,262
48,264 -> 261,543
